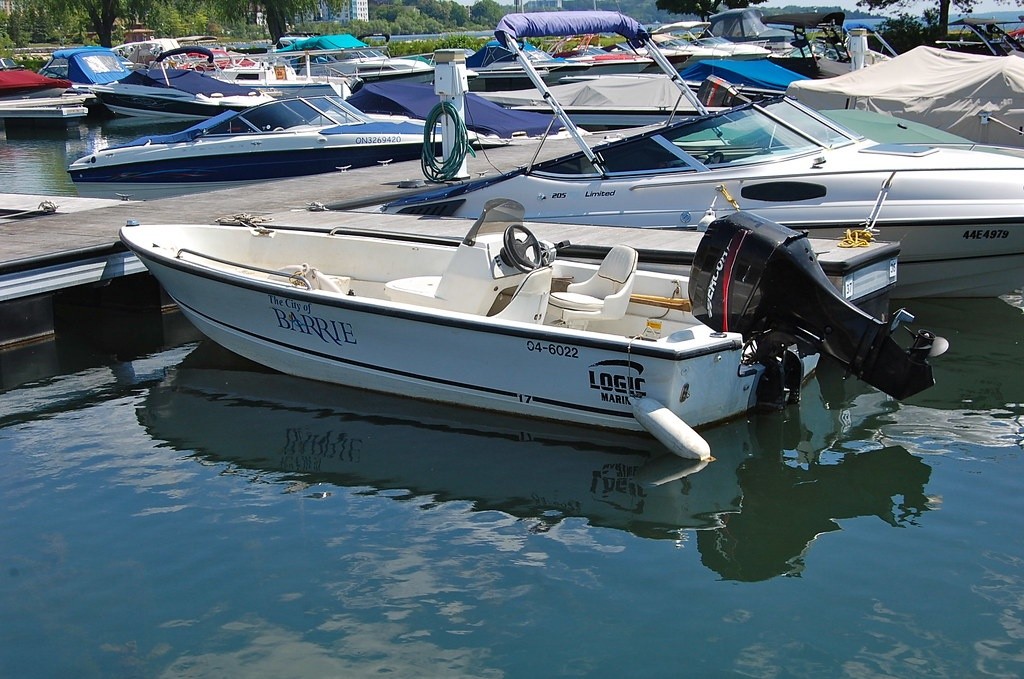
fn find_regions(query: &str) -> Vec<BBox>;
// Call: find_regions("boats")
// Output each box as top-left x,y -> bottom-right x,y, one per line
432,11 -> 1024,304
0,5 -> 890,203
782,15 -> 1023,151
117,199 -> 949,461
135,335 -> 938,583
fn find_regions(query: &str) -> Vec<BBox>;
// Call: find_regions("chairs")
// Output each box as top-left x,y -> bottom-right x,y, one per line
492,267 -> 554,325
551,246 -> 638,329
